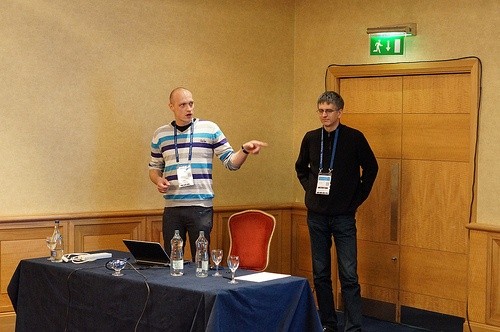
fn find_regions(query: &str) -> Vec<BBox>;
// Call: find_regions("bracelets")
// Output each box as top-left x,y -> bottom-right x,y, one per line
241,146 -> 250,154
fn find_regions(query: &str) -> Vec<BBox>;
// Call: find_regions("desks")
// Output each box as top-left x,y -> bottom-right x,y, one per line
7,249 -> 324,332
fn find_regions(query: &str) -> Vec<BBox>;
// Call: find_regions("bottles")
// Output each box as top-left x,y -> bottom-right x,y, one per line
194,230 -> 209,278
169,230 -> 184,276
50,220 -> 63,263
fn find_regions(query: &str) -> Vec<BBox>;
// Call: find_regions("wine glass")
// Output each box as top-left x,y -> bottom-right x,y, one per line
109,260 -> 126,277
227,255 -> 239,285
211,249 -> 224,277
46,236 -> 56,260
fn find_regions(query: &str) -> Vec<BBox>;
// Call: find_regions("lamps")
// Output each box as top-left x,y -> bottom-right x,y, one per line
366,22 -> 417,37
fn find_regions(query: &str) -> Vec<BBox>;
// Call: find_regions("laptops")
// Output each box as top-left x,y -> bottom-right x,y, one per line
123,238 -> 190,266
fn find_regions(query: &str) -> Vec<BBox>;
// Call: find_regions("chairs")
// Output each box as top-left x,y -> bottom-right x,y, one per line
227,210 -> 276,271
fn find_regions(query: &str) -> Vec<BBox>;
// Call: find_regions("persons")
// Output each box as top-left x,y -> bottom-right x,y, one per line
149,87 -> 268,264
295,91 -> 378,332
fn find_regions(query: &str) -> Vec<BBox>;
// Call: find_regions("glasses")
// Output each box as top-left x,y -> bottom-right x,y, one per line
316,108 -> 339,114
